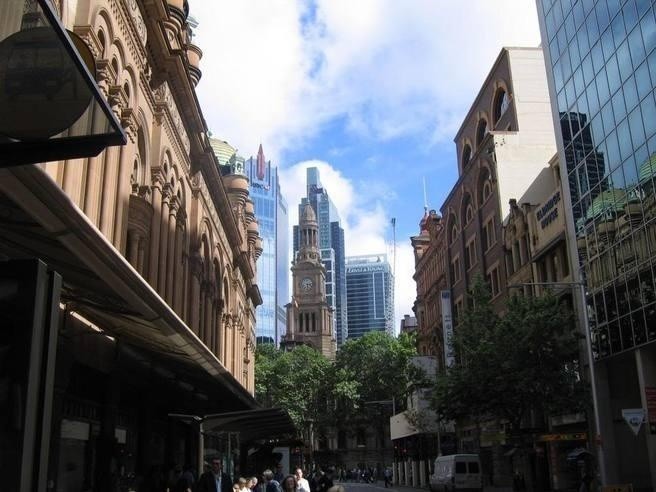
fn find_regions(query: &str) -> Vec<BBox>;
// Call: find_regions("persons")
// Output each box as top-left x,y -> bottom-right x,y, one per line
111,454 -> 393,492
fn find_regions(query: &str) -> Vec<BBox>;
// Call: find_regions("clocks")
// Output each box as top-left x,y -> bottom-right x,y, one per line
301,276 -> 314,293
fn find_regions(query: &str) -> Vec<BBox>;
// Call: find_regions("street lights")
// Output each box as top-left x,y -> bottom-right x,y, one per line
505,278 -> 607,484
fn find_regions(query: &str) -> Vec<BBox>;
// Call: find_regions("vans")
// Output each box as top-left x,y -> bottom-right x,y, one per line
430,452 -> 485,492
